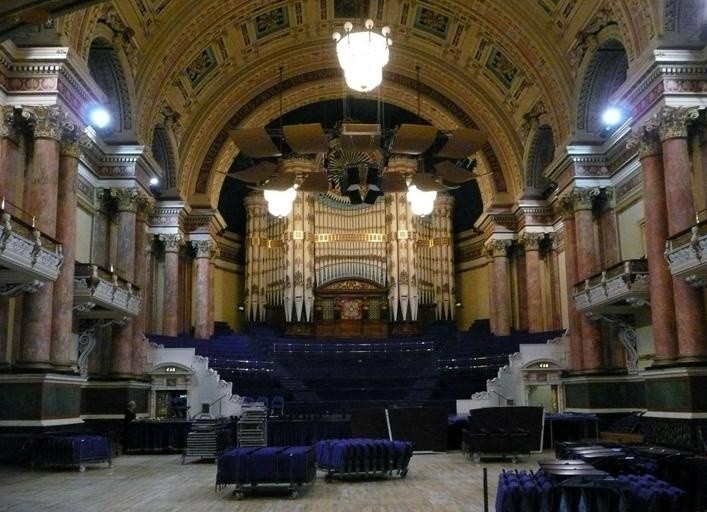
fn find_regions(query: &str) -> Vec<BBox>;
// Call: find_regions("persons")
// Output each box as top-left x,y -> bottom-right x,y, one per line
126,401 -> 137,422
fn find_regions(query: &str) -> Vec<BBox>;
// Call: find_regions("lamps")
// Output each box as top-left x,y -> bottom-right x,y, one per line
406,181 -> 437,217
332,1 -> 392,93
213,67 -> 492,206
264,184 -> 299,219
